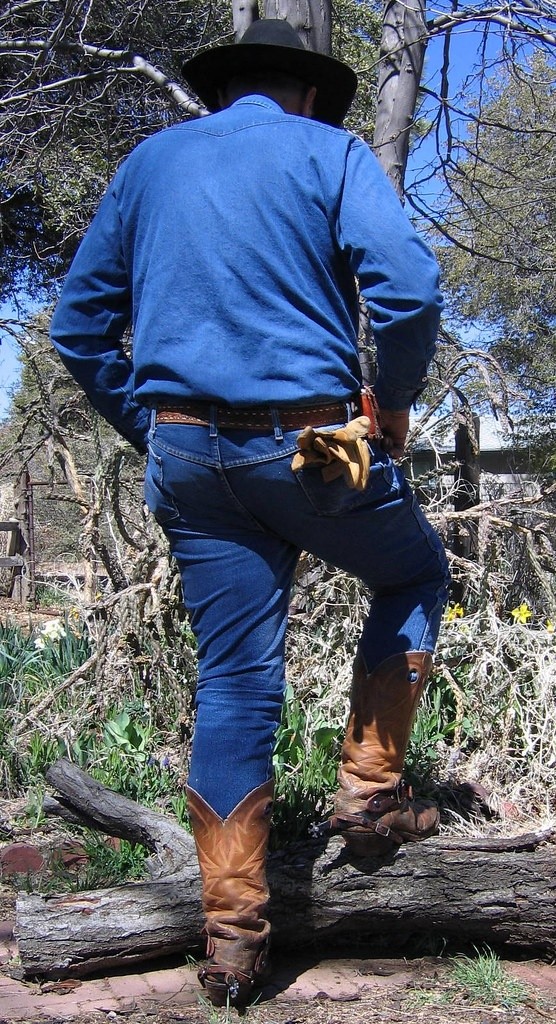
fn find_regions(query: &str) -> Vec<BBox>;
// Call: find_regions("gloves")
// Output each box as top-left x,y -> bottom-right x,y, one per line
291,415 -> 371,491
377,408 -> 409,458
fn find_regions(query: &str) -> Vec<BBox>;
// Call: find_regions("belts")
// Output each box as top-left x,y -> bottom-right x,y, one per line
149,399 -> 361,429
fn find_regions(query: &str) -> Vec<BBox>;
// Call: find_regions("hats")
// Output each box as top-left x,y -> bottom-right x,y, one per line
181,19 -> 358,128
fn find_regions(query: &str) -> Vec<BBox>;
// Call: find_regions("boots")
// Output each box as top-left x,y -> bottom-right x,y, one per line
328,645 -> 441,857
184,775 -> 276,1007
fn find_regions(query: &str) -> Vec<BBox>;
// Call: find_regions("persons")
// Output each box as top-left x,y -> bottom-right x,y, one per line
48,18 -> 452,1006
